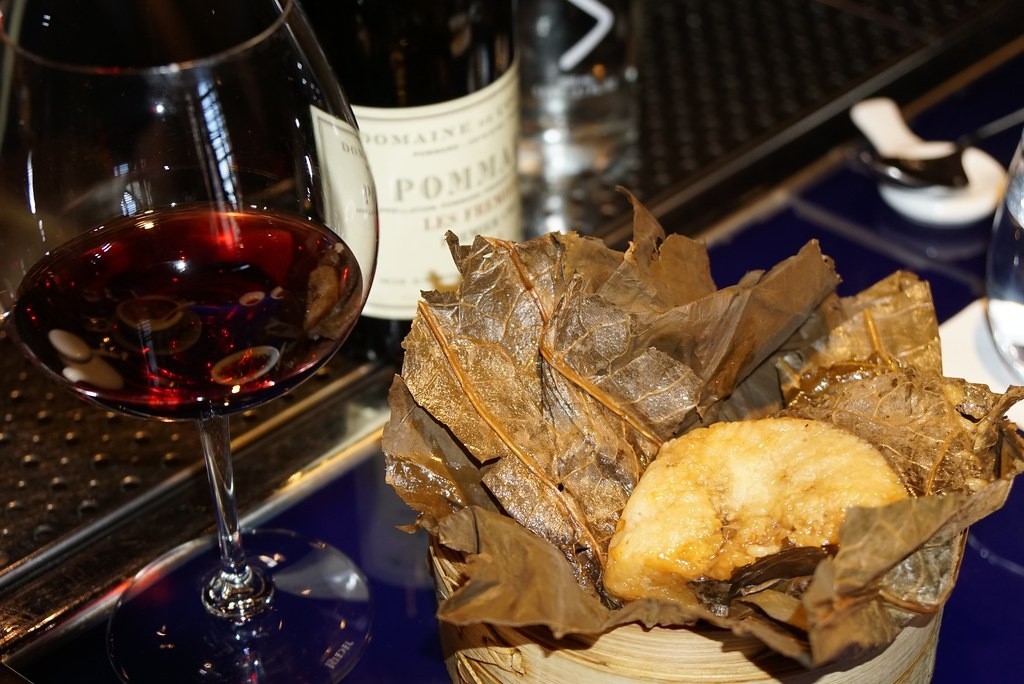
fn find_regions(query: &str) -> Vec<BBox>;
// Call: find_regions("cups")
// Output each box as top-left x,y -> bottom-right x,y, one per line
510,0 -> 646,240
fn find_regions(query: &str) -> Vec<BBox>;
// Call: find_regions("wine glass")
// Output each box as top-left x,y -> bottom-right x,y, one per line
0,0 -> 379,684
965,129 -> 1023,572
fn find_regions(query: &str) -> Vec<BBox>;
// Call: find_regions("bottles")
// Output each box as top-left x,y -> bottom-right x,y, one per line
286,0 -> 522,367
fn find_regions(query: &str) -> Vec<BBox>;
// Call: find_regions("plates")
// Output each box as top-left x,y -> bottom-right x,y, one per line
937,292 -> 1024,431
